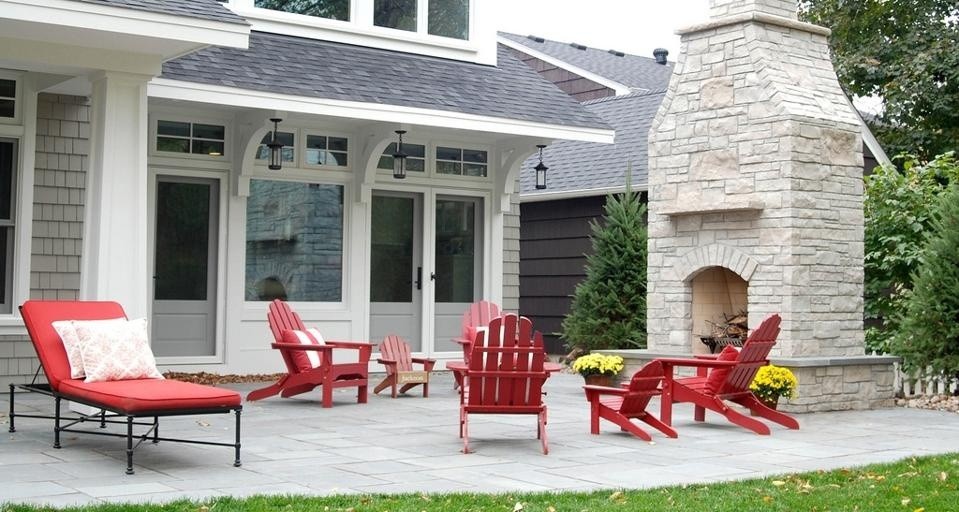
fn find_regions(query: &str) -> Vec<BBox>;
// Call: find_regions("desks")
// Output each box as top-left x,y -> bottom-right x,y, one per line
446,361 -> 561,404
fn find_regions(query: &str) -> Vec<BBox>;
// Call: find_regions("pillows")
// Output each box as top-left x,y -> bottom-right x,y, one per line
71,318 -> 166,383
703,344 -> 740,396
281,323 -> 327,372
51,318 -> 86,380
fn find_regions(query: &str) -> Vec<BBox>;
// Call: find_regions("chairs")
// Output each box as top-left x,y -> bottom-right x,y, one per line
657,313 -> 800,434
247,299 -> 378,408
582,360 -> 678,442
10,300 -> 243,474
374,335 -> 437,399
460,312 -> 551,454
452,300 -> 508,361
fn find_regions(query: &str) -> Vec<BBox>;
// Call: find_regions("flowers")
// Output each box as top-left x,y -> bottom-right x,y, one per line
750,365 -> 800,405
572,353 -> 624,376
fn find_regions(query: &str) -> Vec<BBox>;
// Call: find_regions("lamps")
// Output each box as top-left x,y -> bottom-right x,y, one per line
534,144 -> 549,189
267,118 -> 285,170
392,130 -> 408,179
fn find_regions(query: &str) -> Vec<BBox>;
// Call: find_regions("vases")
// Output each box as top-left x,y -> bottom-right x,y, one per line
585,374 -> 611,387
756,394 -> 780,411
